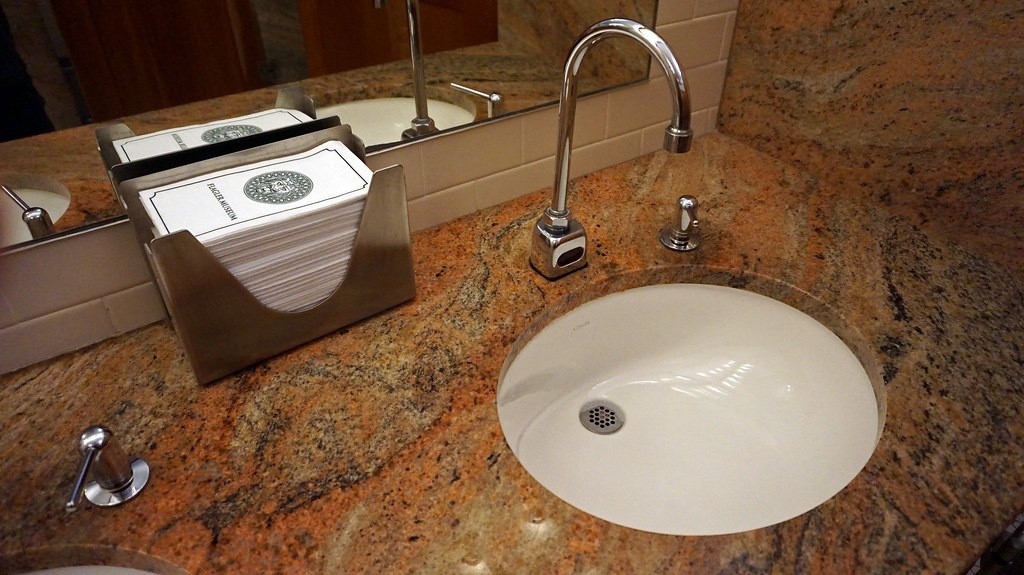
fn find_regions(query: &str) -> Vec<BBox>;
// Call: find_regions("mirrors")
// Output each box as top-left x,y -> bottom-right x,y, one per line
0,0 -> 661,252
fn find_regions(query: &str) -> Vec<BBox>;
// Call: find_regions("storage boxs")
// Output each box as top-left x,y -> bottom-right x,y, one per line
99,80 -> 344,212
121,126 -> 426,388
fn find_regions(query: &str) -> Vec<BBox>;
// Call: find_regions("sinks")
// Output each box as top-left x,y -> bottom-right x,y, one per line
496,258 -> 886,539
309,84 -> 480,146
0,546 -> 194,575
0,169 -> 73,248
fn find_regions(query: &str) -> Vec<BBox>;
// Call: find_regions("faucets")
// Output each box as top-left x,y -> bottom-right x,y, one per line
376,0 -> 443,140
528,16 -> 693,279
